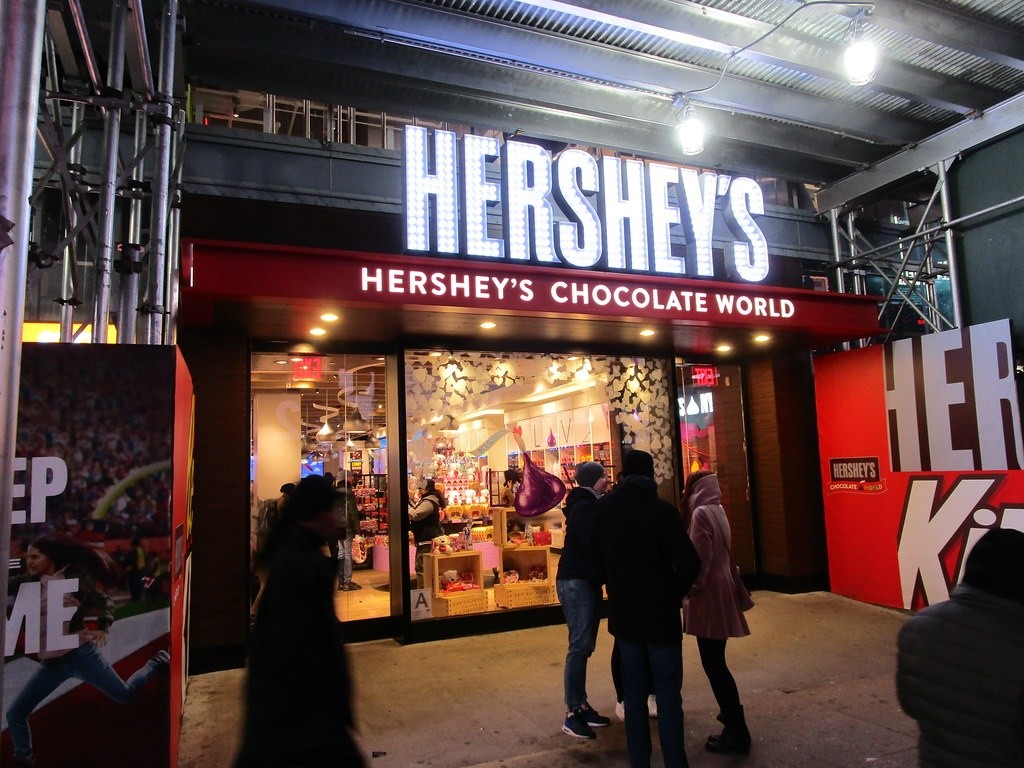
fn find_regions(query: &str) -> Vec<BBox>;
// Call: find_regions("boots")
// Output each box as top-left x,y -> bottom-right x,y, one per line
706,704 -> 751,756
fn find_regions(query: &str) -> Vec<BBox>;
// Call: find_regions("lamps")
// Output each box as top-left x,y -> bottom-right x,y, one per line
364,399 -> 380,449
675,104 -> 705,156
346,433 -> 355,446
301,450 -> 310,464
312,449 -> 324,462
341,439 -> 357,454
324,444 -> 339,458
342,370 -> 371,433
317,388 -> 336,444
842,21 -> 878,88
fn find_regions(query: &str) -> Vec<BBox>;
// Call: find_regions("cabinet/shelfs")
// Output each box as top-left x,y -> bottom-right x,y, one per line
467,425 -> 711,508
351,474 -> 388,571
498,546 -> 556,587
496,583 -> 553,608
493,508 -> 548,547
423,549 -> 489,598
432,590 -> 488,616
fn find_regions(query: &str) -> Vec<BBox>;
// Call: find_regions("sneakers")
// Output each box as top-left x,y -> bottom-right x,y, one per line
581,705 -> 610,727
561,712 -> 595,739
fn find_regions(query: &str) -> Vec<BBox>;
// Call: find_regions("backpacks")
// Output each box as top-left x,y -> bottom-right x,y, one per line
259,496 -> 284,536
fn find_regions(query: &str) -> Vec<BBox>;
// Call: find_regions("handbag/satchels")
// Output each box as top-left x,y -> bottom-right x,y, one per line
732,576 -> 754,612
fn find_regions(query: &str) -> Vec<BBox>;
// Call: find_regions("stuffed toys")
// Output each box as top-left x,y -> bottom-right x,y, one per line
466,468 -> 478,481
443,570 -> 458,582
448,491 -> 462,505
433,454 -> 446,467
465,490 -> 479,504
450,463 -> 463,478
433,535 -> 452,556
480,489 -> 490,503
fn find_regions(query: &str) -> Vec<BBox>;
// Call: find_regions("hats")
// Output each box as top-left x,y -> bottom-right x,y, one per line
575,461 -> 604,490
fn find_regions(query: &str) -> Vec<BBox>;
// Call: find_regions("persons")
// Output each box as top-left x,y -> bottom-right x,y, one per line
589,450 -> 690,768
895,526 -> 1024,768
503,469 -> 520,489
0,374 -> 177,768
679,470 -> 752,754
556,462 -> 610,738
407,479 -> 445,576
238,465 -> 365,768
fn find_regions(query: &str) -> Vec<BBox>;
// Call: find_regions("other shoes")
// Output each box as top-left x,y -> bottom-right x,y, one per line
152,651 -> 170,666
647,692 -> 659,715
616,701 -> 625,723
337,581 -> 361,591
